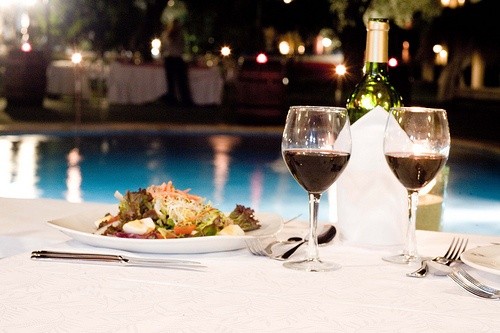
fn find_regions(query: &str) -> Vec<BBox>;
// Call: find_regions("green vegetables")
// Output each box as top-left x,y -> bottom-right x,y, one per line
98,188 -> 262,237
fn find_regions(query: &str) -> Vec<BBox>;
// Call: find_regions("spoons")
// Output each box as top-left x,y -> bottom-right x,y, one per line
280,223 -> 336,259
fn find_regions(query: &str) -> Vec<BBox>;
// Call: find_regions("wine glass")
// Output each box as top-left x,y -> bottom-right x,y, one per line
280,106 -> 352,269
382,108 -> 451,263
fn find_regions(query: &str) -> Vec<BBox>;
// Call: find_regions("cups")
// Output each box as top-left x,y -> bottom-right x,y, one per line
413,166 -> 448,232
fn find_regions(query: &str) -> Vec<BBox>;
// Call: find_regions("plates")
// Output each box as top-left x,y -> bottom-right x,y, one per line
47,217 -> 283,253
460,244 -> 500,276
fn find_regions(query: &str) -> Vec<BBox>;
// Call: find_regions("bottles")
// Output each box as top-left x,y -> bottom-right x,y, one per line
347,18 -> 405,128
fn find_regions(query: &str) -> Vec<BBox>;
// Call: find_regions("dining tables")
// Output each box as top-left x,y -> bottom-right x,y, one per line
46,60 -> 168,105
187,63 -> 224,104
0,196 -> 500,331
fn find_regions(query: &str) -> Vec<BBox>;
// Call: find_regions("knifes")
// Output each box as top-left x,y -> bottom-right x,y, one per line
31,251 -> 207,269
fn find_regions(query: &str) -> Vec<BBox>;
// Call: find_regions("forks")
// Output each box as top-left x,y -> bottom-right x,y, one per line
407,236 -> 467,277
243,234 -> 295,255
448,269 -> 500,299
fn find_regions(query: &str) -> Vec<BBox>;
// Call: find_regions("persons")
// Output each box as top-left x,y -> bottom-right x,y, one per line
160,18 -> 194,106
